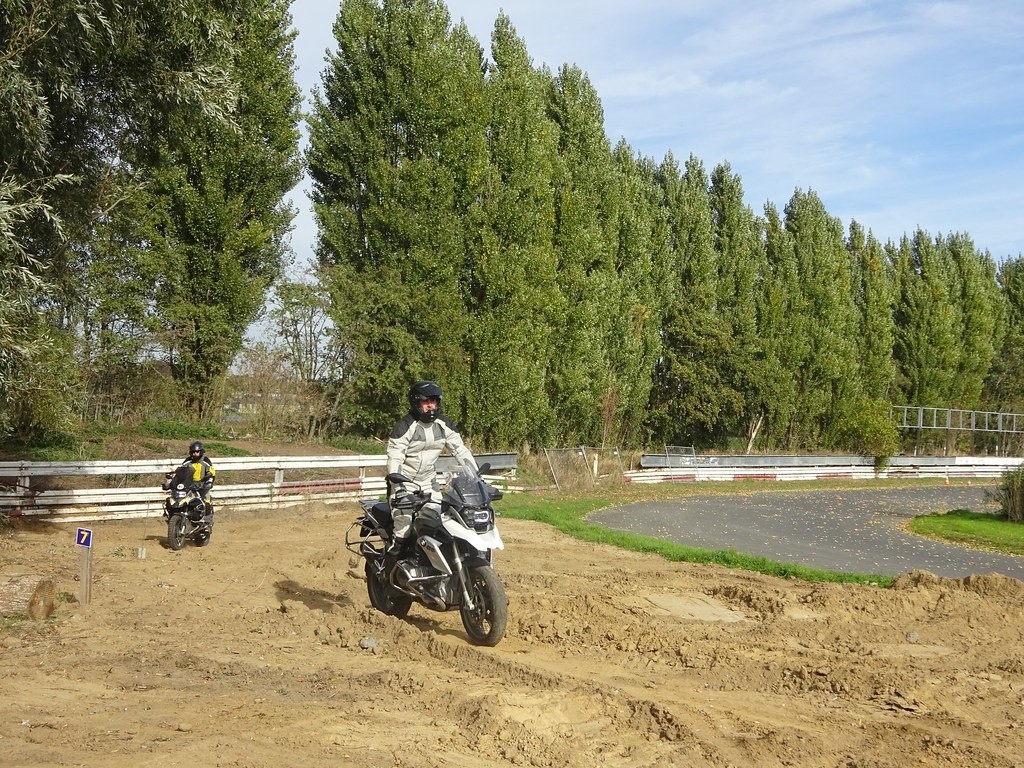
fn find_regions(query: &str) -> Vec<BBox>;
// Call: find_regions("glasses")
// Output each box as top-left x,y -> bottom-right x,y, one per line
192,450 -> 200,453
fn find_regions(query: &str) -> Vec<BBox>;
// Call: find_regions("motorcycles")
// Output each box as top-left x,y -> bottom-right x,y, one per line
163,472 -> 214,551
345,462 -> 508,647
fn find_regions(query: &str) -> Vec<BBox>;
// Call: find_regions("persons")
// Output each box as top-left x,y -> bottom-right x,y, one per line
383,380 -> 485,585
160,441 -> 217,534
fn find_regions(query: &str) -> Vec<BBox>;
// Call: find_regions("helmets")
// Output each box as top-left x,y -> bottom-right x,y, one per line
190,442 -> 203,461
408,382 -> 441,422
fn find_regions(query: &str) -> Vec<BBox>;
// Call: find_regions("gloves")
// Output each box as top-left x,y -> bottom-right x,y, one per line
204,482 -> 213,489
484,482 -> 499,497
394,490 -> 415,505
162,482 -> 170,490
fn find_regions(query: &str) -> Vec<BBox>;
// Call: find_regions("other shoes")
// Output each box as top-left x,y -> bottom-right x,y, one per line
377,570 -> 399,598
